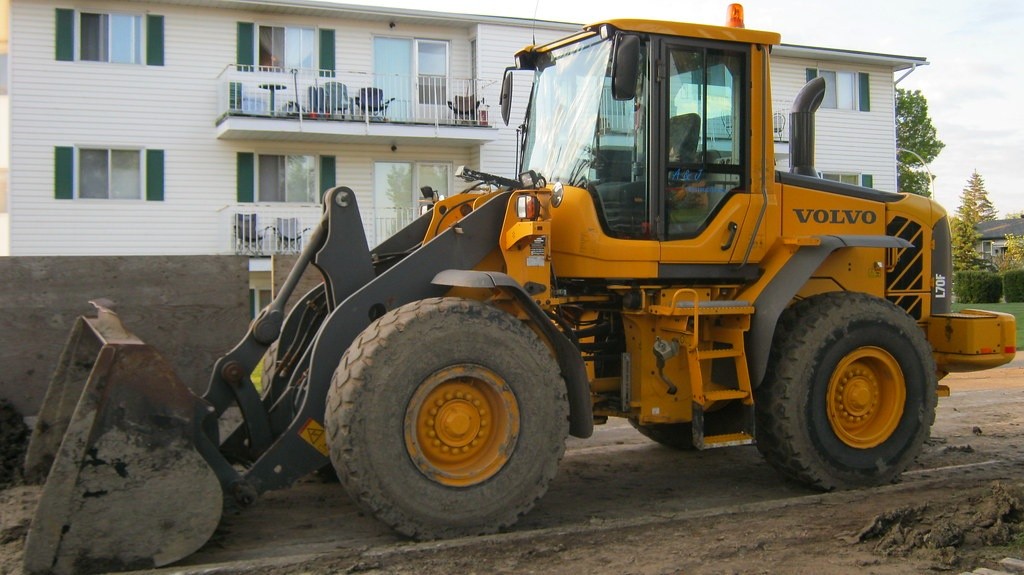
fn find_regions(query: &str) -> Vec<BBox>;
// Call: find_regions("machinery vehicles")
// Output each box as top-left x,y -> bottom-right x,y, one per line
19,1 -> 1017,574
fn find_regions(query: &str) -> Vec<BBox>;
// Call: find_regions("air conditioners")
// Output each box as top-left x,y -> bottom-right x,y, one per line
216,78 -> 243,120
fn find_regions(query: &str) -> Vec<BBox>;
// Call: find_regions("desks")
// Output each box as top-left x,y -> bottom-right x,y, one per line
259,84 -> 287,116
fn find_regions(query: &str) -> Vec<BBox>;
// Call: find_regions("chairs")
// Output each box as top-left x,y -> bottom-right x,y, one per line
355,88 -> 395,120
273,217 -> 311,254
309,86 -> 330,112
596,113 -> 701,223
325,82 -> 354,121
234,213 -> 272,255
448,94 -> 480,124
